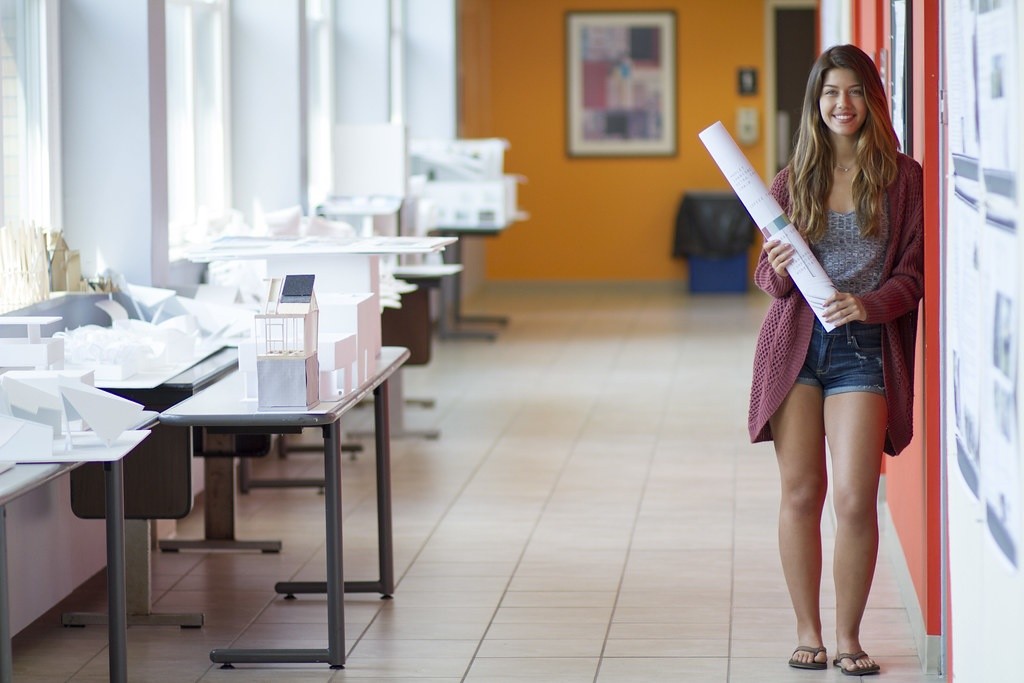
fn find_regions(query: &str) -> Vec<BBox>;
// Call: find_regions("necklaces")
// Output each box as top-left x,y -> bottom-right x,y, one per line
834,164 -> 854,171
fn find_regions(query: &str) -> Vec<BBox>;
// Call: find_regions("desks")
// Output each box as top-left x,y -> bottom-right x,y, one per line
63,347 -> 282,631
427,210 -> 528,342
0,410 -> 163,683
188,228 -> 458,294
156,343 -> 411,669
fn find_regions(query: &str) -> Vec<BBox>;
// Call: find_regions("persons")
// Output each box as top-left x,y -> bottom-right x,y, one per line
747,44 -> 925,676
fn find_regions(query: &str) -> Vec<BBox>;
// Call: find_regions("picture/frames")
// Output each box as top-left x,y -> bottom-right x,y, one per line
564,11 -> 677,159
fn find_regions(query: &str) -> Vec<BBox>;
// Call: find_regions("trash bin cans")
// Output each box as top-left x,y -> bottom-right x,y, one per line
684,191 -> 750,294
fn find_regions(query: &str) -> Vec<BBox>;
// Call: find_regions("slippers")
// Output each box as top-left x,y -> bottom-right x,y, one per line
788,645 -> 827,670
833,651 -> 880,676
